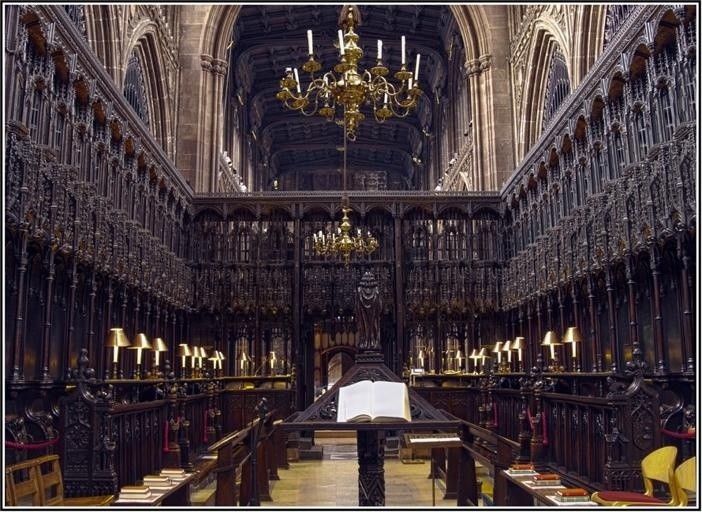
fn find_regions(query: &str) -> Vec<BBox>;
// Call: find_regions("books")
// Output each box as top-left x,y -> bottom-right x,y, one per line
508,464 -> 589,503
336,380 -> 412,424
118,467 -> 186,501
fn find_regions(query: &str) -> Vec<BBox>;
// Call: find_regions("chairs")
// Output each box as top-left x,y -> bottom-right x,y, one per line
591,446 -> 696,506
5,455 -> 116,505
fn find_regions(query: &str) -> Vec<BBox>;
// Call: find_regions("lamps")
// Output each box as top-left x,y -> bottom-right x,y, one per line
410,326 -> 583,366
274,5 -> 424,271
102,326 -> 279,377
